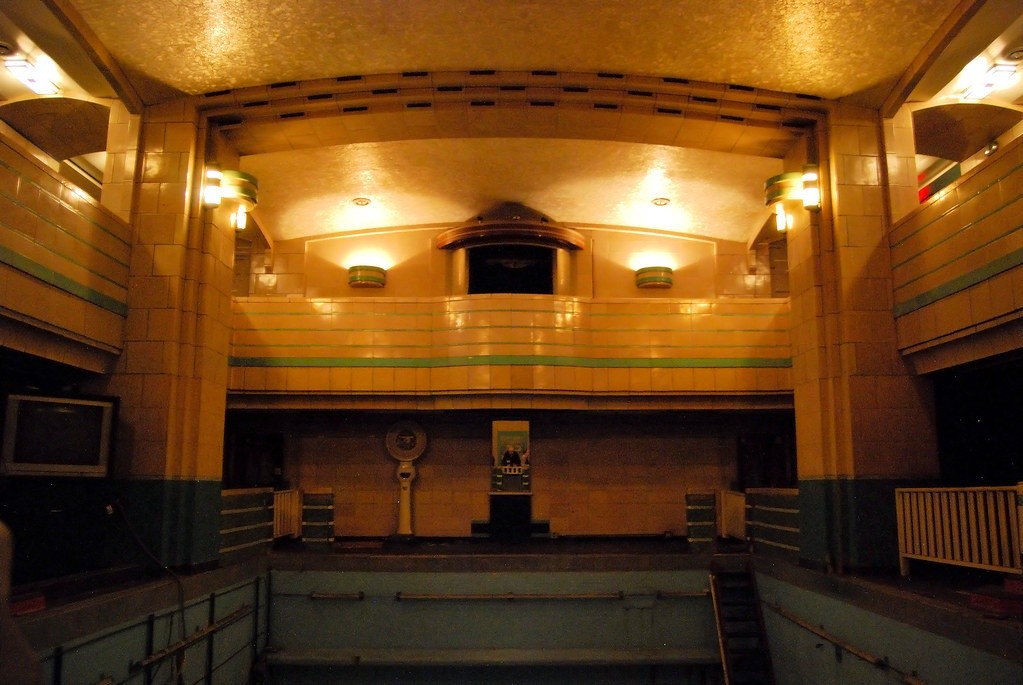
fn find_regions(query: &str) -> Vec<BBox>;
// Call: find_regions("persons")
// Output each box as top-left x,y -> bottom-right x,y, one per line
0,501 -> 49,685
502,445 -> 520,466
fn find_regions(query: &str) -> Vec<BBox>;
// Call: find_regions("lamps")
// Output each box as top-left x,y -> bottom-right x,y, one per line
203,160 -> 221,207
764,173 -> 802,214
963,63 -> 1019,100
347,266 -> 385,289
637,267 -> 673,287
220,170 -> 259,210
801,164 -> 822,212
5,57 -> 59,97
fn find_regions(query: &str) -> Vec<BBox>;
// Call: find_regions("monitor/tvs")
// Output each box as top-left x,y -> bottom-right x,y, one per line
1,396 -> 114,477
